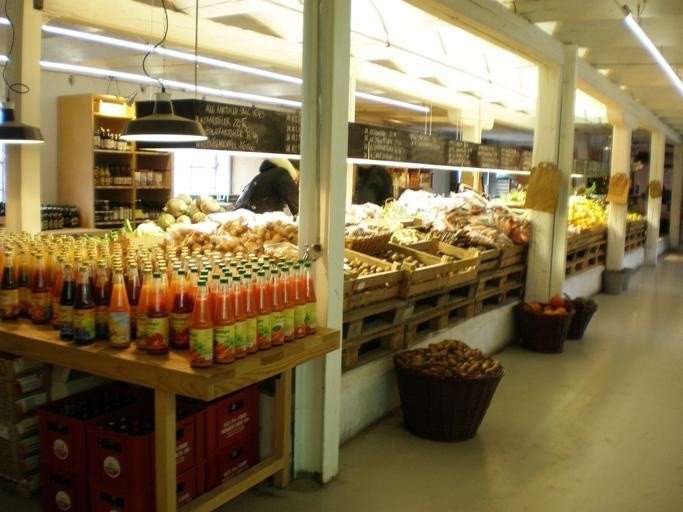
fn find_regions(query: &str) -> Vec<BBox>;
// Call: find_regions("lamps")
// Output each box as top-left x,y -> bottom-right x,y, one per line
615,0 -> 683,99
115,0 -> 209,149
0,1 -> 45,148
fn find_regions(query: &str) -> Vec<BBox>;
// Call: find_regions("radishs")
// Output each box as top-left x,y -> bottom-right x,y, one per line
158,194 -> 220,231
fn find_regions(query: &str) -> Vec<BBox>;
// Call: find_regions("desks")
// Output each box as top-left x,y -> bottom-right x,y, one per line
39,227 -> 111,238
0,309 -> 342,512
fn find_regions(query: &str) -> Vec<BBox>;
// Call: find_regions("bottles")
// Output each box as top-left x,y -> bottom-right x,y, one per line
188,263 -> 316,369
95,199 -> 165,223
1,229 -> 304,354
39,203 -> 80,230
93,127 -> 128,152
45,391 -> 192,454
92,163 -> 162,187
0,201 -> 5,227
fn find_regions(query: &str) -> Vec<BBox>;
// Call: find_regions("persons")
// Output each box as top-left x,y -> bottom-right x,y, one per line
234,157 -> 298,217
355,165 -> 389,204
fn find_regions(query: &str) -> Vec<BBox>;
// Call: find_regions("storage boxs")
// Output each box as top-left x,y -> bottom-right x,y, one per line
289,203 -> 526,308
35,380 -> 262,510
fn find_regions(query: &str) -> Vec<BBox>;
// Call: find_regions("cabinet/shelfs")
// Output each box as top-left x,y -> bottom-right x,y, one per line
55,91 -> 172,235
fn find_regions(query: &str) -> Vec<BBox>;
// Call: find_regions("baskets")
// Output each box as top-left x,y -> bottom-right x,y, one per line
393,347 -> 504,442
513,301 -> 577,353
568,311 -> 594,339
